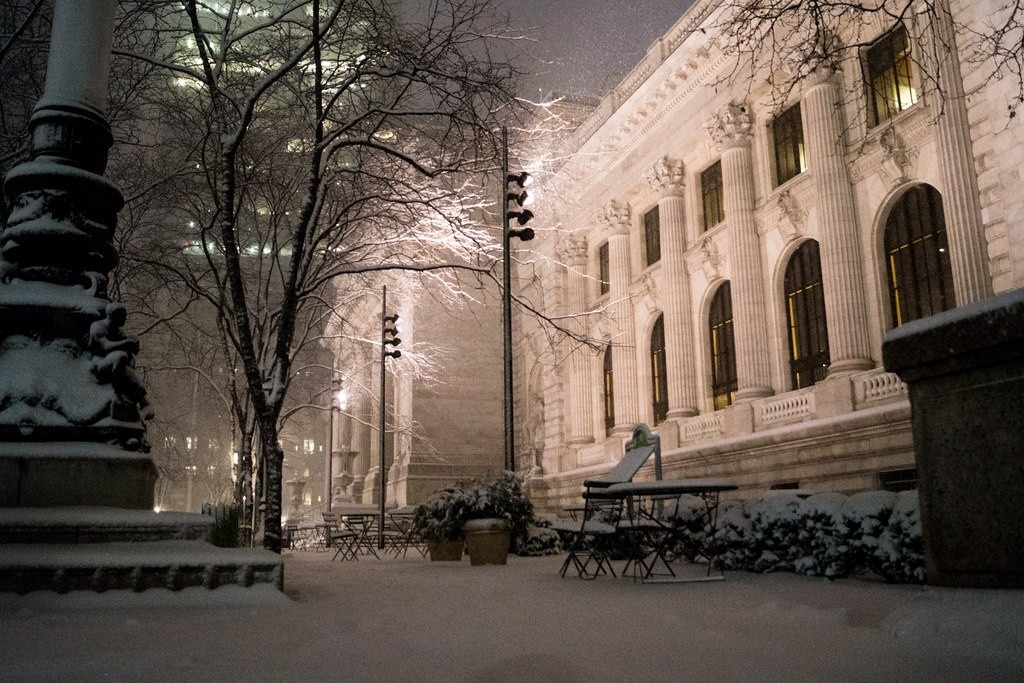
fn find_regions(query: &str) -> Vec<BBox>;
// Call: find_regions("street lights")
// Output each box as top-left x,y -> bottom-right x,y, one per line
499,123 -> 535,479
378,284 -> 402,548
325,368 -> 343,511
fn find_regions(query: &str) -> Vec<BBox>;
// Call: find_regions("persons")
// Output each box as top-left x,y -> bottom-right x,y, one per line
88,302 -> 156,420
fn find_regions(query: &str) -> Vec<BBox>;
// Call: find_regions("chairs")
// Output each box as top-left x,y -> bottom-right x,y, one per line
287,511 -> 428,564
605,481 -> 680,583
550,480 -> 625,579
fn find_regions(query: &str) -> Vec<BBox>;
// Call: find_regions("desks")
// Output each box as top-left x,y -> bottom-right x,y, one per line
605,480 -> 739,584
